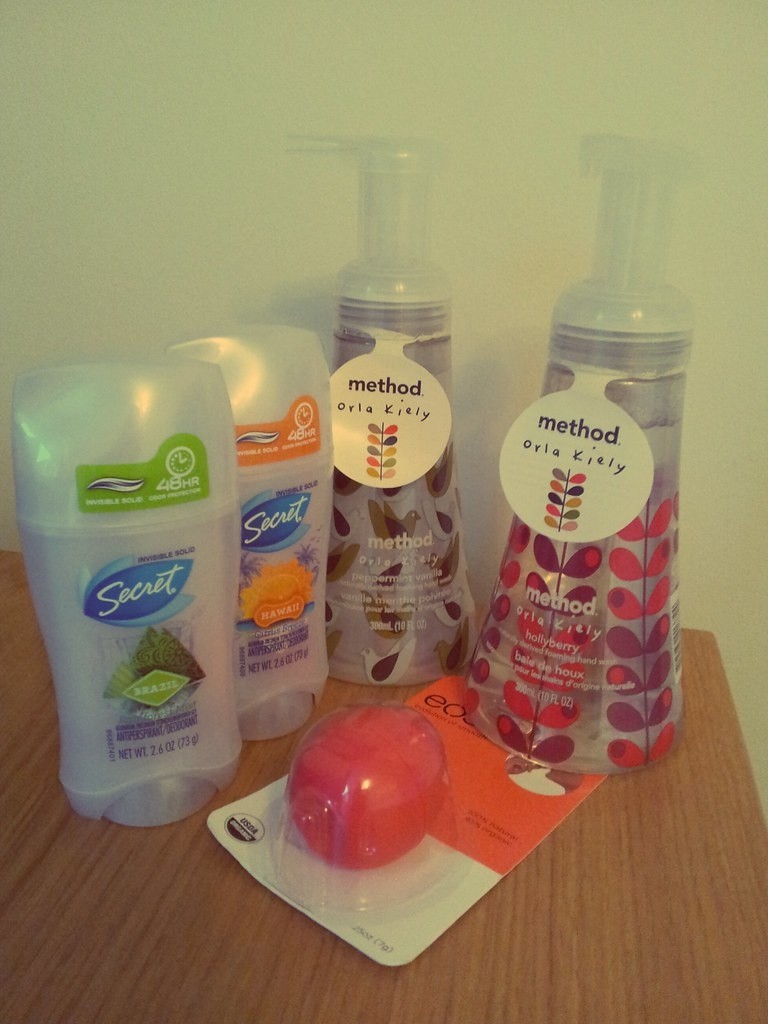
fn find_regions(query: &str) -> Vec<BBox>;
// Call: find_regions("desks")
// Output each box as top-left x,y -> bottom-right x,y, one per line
0,547 -> 768,1023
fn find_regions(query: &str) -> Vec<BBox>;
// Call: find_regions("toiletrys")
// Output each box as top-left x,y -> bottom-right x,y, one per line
278,130 -> 480,690
461,135 -> 706,773
11,354 -> 244,827
141,320 -> 327,740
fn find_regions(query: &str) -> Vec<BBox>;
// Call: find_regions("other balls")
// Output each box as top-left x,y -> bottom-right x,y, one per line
288,704 -> 447,871
